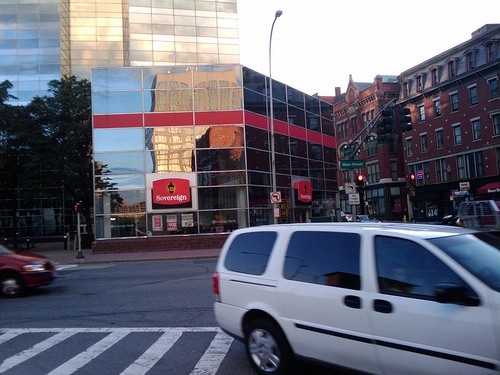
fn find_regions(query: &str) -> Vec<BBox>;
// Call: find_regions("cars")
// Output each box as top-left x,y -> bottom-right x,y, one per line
441,200 -> 500,228
0,244 -> 56,298
348,214 -> 380,222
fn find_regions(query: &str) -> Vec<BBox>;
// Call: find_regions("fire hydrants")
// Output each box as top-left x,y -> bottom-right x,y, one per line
403,215 -> 406,223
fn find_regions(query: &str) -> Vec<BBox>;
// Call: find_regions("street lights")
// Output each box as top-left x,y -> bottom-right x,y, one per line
268,9 -> 284,223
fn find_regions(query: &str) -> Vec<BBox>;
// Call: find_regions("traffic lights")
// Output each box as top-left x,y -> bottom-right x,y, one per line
399,107 -> 413,133
356,174 -> 366,195
408,172 -> 415,182
74,200 -> 83,213
382,109 -> 395,134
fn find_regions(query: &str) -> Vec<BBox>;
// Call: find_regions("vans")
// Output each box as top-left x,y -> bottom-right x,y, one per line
211,223 -> 500,374
